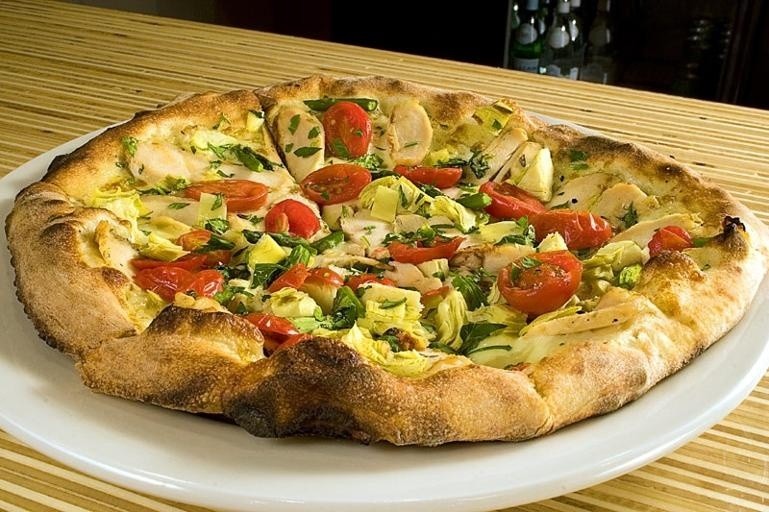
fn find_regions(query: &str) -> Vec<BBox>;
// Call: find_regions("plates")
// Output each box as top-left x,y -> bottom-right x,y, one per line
1,101 -> 768,511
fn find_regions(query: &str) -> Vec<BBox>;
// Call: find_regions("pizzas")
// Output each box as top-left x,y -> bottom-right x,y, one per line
4,74 -> 767,447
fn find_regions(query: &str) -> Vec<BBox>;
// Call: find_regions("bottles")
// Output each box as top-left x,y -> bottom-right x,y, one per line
507,0 -> 621,87
